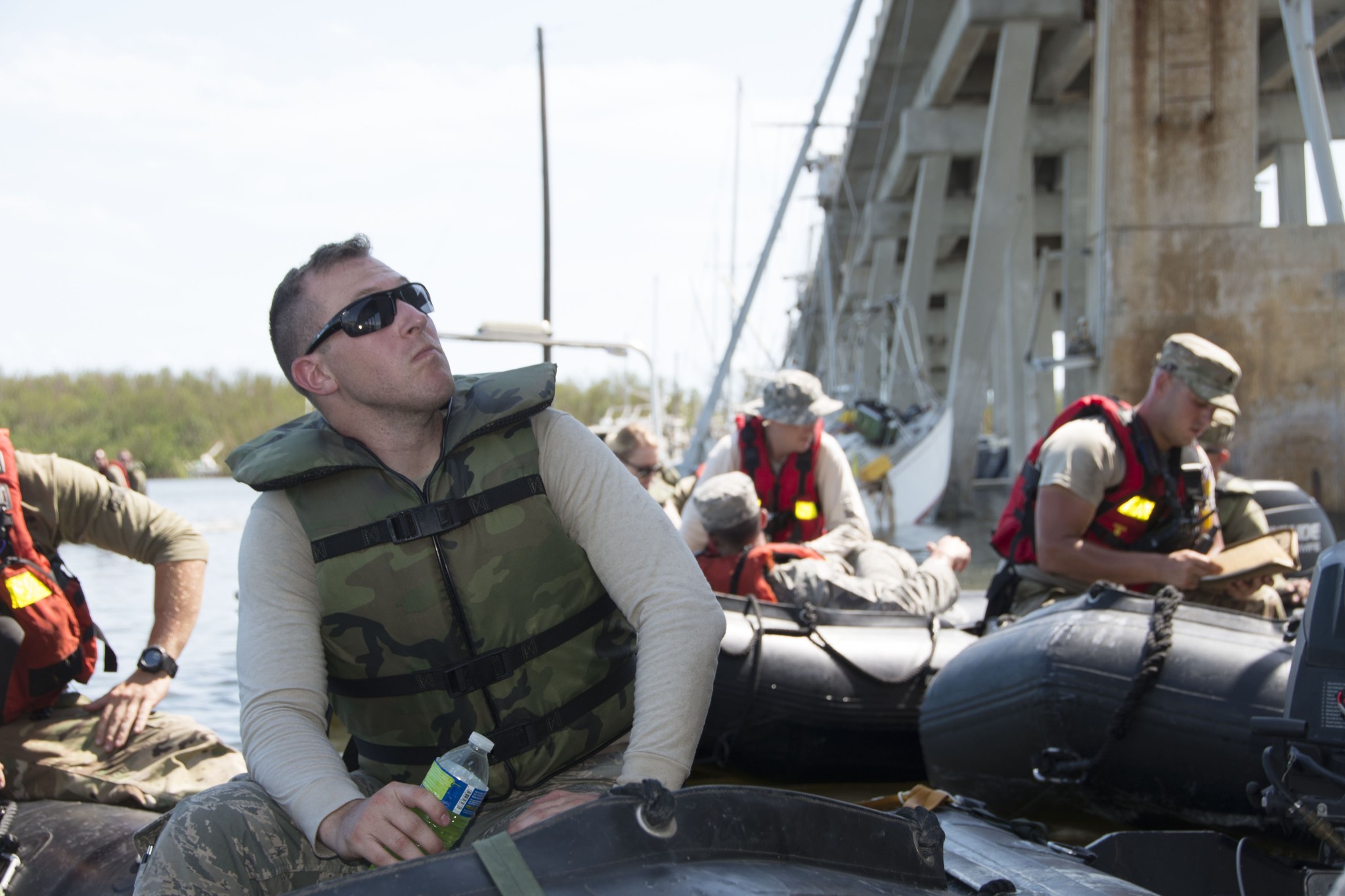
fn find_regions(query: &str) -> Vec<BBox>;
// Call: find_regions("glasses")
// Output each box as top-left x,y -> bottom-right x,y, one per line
624,461 -> 663,476
305,282 -> 435,355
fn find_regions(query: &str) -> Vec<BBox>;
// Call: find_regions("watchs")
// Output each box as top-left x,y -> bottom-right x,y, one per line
137,645 -> 178,678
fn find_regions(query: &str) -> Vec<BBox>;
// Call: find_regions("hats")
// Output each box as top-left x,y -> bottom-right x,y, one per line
693,471 -> 762,529
743,369 -> 844,424
1199,409 -> 1236,443
1156,333 -> 1242,415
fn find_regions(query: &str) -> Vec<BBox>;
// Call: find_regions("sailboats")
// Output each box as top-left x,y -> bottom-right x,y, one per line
432,1 -> 1345,790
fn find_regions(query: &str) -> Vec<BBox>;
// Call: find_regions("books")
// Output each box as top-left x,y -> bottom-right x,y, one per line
1201,529 -> 1302,591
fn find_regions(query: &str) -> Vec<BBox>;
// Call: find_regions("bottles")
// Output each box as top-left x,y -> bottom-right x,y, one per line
368,731 -> 495,871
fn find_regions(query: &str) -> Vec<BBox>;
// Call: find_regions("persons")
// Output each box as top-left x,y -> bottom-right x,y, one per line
91,448 -> 147,495
983,332 -> 1285,636
1198,409 -> 1312,619
693,472 -> 971,618
131,235 -> 727,896
678,367 -> 874,556
0,427 -> 247,810
606,421 -> 680,529
